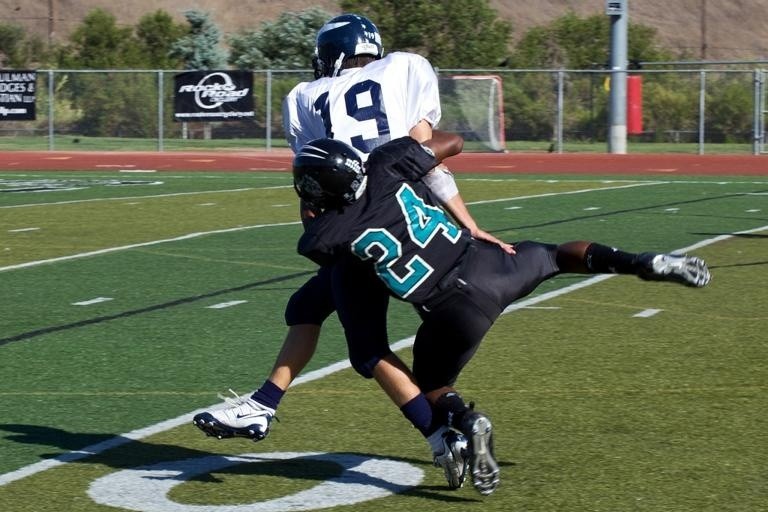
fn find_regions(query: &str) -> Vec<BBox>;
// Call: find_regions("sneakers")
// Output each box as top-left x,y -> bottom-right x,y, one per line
633,251 -> 710,289
428,426 -> 473,491
454,402 -> 501,497
190,388 -> 280,443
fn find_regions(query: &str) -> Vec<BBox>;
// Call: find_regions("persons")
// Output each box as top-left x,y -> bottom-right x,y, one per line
290,128 -> 713,496
191,14 -> 519,491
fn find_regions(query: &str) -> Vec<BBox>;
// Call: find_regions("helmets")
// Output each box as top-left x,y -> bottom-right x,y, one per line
292,136 -> 370,207
313,12 -> 385,78
